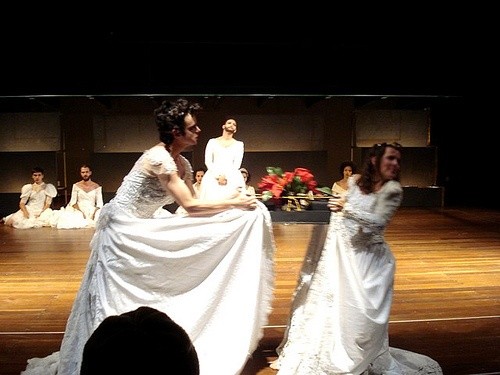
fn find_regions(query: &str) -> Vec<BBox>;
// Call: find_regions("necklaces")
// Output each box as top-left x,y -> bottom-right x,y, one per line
35,182 -> 45,186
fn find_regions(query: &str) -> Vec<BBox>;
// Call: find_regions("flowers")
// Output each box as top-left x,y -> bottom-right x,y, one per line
257,166 -> 319,202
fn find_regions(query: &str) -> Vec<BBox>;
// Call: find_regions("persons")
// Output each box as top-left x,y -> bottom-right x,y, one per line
80,305 -> 200,375
240,168 -> 255,198
62,164 -> 104,229
59,97 -> 256,375
268,141 -> 405,375
332,162 -> 356,198
198,117 -> 245,202
192,168 -> 204,196
0,168 -> 57,230
